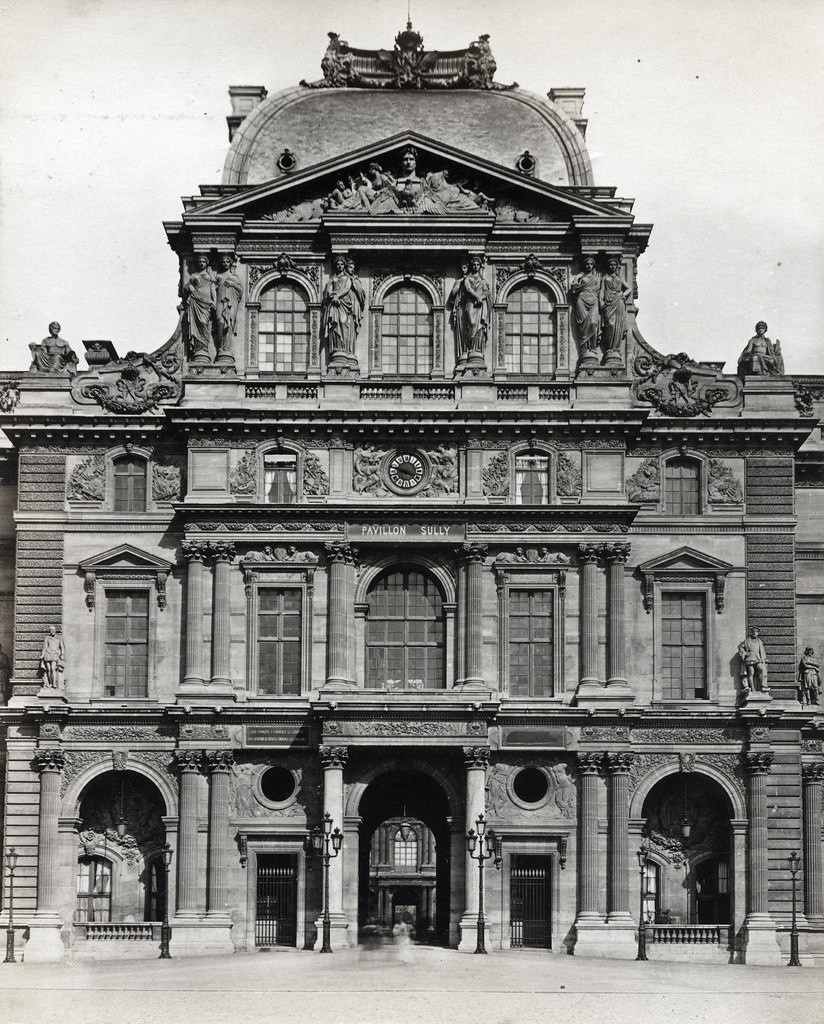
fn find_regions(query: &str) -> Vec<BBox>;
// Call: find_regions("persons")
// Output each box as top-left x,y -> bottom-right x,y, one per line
449,257 -> 492,354
744,322 -> 778,374
485,754 -> 524,819
40,625 -> 66,688
0,643 -> 9,706
328,145 -> 496,218
571,258 -> 631,352
537,754 -> 577,820
186,255 -> 244,353
35,321 -> 79,373
399,22 -> 418,67
322,256 -> 366,355
738,628 -> 770,692
799,646 -> 823,706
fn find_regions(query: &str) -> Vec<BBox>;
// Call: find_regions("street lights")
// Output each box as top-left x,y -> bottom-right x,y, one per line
310,813 -> 343,953
160,842 -> 174,958
5,847 -> 17,963
466,814 -> 497,953
787,851 -> 803,968
636,844 -> 650,960
